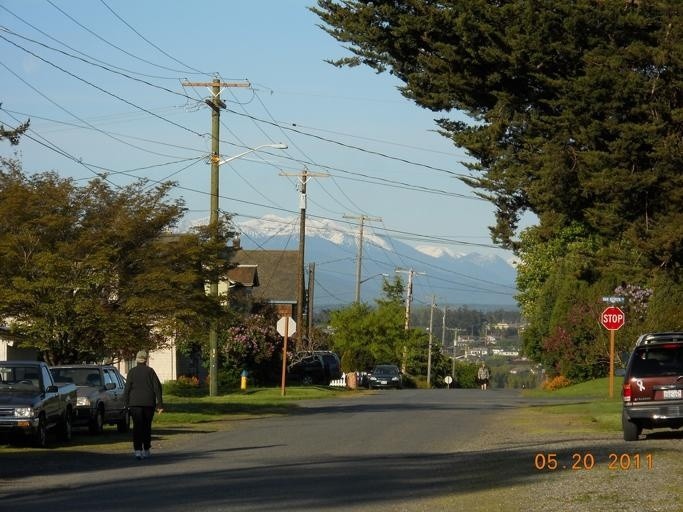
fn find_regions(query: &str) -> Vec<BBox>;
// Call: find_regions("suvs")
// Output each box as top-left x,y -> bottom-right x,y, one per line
620,330 -> 683,442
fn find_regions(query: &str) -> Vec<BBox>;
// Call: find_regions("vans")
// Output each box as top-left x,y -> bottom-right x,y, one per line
287,350 -> 342,385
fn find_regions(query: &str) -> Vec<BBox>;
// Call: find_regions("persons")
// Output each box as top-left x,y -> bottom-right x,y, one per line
123,351 -> 163,460
478,364 -> 489,391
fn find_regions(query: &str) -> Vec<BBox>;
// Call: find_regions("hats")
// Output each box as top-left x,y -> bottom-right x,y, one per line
137,351 -> 148,363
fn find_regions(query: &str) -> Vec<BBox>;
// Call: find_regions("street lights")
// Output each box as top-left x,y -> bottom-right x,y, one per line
208,143 -> 289,398
355,272 -> 389,306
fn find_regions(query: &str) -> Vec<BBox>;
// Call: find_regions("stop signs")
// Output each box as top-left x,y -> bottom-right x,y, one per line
600,307 -> 625,331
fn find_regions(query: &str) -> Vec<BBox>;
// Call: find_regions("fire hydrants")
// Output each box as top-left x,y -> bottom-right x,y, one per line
240,369 -> 249,389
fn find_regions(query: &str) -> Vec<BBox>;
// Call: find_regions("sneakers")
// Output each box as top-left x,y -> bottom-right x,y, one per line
136,450 -> 152,459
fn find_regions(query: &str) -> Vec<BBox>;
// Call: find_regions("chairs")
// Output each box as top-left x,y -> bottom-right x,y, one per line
86,374 -> 99,386
24,373 -> 40,391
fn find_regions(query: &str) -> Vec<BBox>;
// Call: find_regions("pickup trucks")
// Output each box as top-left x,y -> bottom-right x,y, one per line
0,360 -> 130,449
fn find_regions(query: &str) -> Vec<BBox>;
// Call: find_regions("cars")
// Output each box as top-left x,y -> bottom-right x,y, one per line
368,364 -> 404,390
